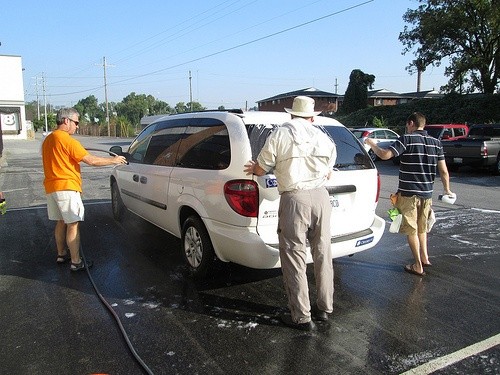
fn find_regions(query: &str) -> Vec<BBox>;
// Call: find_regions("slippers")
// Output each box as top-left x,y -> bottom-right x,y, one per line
403,263 -> 426,276
421,258 -> 433,267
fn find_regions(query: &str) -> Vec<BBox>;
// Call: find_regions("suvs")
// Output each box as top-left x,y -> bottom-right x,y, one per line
423,124 -> 469,142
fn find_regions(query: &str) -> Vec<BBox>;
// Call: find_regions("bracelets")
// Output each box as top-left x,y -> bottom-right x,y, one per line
252,164 -> 256,175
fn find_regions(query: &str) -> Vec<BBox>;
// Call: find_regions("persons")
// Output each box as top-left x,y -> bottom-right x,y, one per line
243,95 -> 337,331
43,108 -> 126,271
0,113 -> 6,214
364,112 -> 452,276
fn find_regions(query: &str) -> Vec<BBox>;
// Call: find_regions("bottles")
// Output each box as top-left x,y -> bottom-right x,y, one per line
438,194 -> 456,205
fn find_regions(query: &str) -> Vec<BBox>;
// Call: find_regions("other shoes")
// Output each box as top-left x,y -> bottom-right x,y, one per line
281,314 -> 312,330
310,303 -> 329,321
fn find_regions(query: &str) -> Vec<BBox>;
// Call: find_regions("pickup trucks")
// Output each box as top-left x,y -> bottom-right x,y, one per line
440,123 -> 500,176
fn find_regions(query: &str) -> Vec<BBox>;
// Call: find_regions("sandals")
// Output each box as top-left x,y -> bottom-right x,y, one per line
56,253 -> 71,264
68,259 -> 93,272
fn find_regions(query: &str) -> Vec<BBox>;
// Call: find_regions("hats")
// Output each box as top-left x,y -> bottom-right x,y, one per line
284,96 -> 322,118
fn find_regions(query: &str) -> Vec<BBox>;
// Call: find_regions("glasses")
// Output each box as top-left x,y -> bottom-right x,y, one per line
63,116 -> 79,127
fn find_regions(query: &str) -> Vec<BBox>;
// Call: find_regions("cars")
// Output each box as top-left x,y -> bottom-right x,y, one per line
108,107 -> 387,278
349,126 -> 403,165
